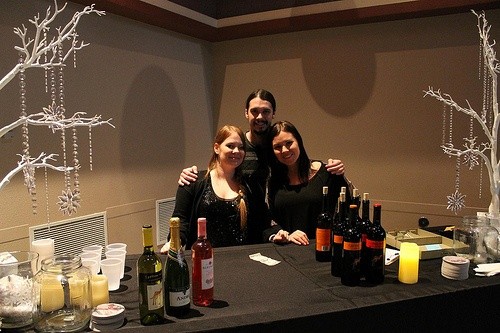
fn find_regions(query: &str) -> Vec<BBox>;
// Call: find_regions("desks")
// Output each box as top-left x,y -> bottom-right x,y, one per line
0,237 -> 500,333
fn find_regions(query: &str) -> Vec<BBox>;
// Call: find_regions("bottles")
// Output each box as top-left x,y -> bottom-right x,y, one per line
31,254 -> 93,333
164,216 -> 190,317
316,186 -> 387,287
191,218 -> 214,307
136,223 -> 164,327
453,216 -> 500,268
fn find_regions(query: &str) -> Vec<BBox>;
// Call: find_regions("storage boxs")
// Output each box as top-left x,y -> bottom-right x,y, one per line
421,225 -> 477,253
386,228 -> 470,260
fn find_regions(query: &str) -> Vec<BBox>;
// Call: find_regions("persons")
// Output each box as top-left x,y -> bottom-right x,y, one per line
177,88 -> 345,188
267,120 -> 351,245
161,126 -> 291,255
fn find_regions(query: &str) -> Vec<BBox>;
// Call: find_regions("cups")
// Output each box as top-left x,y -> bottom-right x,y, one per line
78,242 -> 128,291
0,251 -> 40,332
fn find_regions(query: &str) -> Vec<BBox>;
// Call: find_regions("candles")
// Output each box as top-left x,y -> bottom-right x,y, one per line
398,242 -> 419,284
